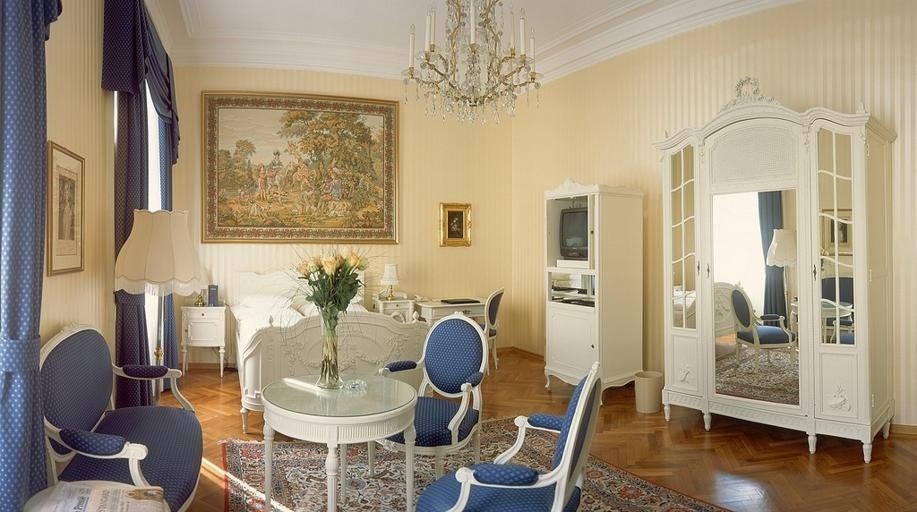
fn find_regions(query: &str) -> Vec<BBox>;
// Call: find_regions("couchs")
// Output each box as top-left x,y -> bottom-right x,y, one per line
240,312 -> 432,433
673,282 -> 743,355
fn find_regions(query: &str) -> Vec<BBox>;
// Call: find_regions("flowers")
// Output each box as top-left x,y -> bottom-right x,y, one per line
278,247 -> 373,389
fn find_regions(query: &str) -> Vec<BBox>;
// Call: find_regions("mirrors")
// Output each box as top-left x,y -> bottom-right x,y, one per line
669,145 -> 697,329
710,187 -> 799,408
819,128 -> 855,344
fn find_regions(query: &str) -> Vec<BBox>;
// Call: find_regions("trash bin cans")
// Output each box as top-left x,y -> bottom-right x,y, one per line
635,372 -> 663,414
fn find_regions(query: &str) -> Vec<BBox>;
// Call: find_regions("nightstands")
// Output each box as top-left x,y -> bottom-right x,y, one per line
181,296 -> 226,378
373,290 -> 414,324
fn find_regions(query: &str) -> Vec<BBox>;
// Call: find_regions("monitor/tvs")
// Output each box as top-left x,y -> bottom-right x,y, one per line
558,208 -> 588,260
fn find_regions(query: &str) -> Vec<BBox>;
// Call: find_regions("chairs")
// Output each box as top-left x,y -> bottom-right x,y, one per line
416,361 -> 602,512
366,312 -> 489,481
38,323 -> 202,512
473,287 -> 506,377
731,286 -> 796,374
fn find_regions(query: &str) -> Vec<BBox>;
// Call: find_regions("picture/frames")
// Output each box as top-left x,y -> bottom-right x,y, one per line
200,91 -> 400,244
440,203 -> 472,247
821,207 -> 854,258
46,140 -> 86,279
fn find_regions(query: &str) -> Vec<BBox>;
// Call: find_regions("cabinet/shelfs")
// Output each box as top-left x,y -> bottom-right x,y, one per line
653,77 -> 899,463
544,182 -> 644,405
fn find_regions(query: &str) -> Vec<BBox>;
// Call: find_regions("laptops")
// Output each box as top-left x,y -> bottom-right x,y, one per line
441,298 -> 481,304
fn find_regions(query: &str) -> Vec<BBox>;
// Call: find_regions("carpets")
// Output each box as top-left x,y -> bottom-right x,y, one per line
717,345 -> 800,405
223,413 -> 734,512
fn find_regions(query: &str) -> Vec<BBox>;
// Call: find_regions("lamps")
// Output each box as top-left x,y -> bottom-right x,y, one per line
766,229 -> 799,336
381,264 -> 400,300
115,209 -> 208,408
405,2 -> 541,124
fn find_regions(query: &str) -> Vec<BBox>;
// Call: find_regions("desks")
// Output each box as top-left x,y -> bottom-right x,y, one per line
416,298 -> 486,325
790,298 -> 852,344
22,480 -> 171,512
261,373 -> 417,512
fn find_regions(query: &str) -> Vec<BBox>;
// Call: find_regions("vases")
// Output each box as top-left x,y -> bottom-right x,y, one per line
316,306 -> 342,389
